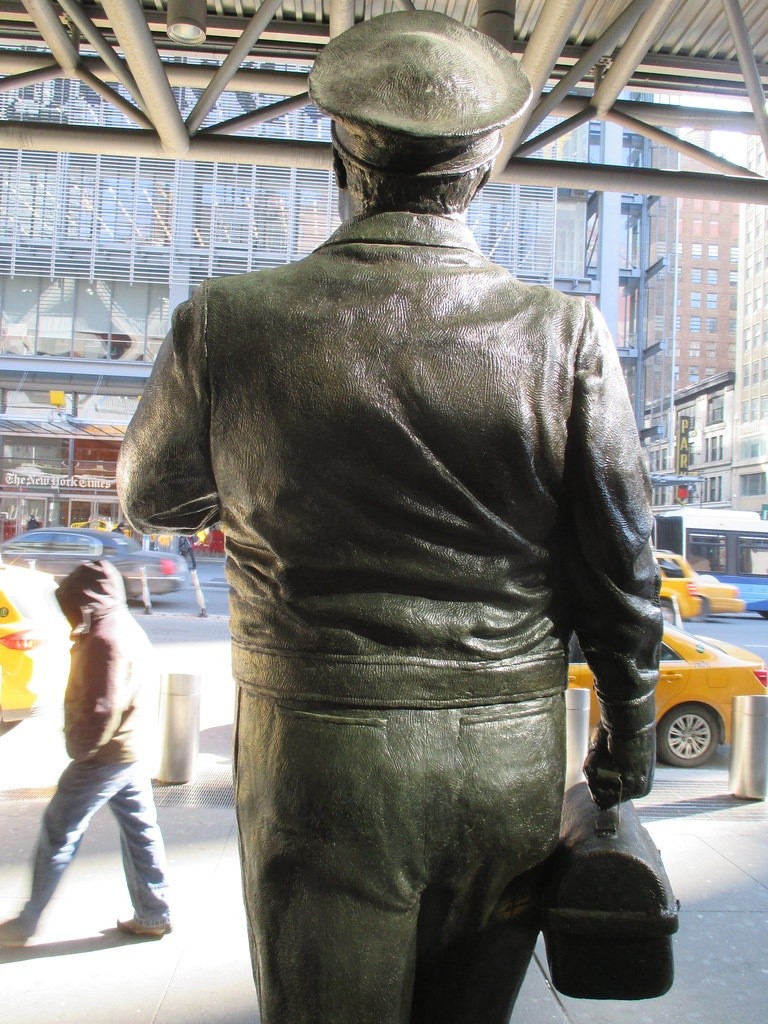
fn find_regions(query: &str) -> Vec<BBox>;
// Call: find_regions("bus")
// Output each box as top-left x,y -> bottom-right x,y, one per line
654,509 -> 768,618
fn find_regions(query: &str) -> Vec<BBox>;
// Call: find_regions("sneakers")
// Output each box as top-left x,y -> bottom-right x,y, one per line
2,920 -> 41,948
116,919 -> 173,938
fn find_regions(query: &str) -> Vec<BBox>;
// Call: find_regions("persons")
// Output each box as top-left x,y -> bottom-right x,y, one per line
1,559 -> 170,946
116,10 -> 664,1021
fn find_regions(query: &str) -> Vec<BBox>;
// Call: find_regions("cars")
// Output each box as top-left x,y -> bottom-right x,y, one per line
571,605 -> 768,767
0,527 -> 188,598
650,550 -> 744,618
0,564 -> 68,724
